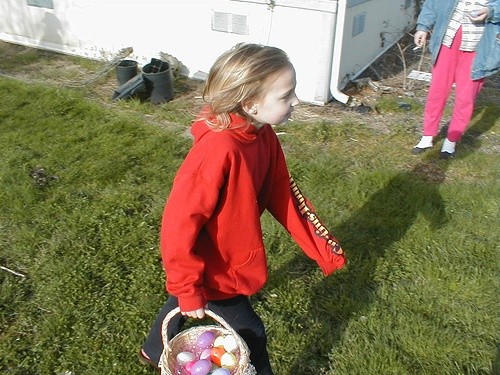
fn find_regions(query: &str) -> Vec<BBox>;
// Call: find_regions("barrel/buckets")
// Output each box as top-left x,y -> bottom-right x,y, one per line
116,59 -> 137,87
143,60 -> 174,105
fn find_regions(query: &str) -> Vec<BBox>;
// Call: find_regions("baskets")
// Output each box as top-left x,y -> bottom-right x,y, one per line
160,307 -> 257,375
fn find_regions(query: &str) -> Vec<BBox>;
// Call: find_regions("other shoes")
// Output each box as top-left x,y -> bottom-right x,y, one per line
138,346 -> 161,375
412,144 -> 432,155
440,147 -> 456,160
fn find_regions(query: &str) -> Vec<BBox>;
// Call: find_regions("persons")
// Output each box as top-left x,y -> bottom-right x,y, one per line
414,1 -> 500,157
137,43 -> 348,375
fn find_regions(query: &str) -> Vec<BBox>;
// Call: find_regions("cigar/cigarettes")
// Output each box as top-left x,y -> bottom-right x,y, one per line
412,45 -> 422,51
